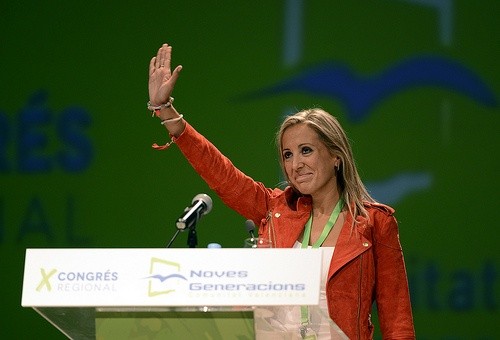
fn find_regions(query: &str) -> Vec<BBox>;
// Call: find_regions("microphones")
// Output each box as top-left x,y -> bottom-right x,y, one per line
245,220 -> 256,245
175,193 -> 213,232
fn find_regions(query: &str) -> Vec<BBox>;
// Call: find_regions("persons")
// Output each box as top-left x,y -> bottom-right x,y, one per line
146,43 -> 416,340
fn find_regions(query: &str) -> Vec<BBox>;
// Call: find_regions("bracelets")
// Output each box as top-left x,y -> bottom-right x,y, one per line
161,113 -> 183,125
147,96 -> 174,118
